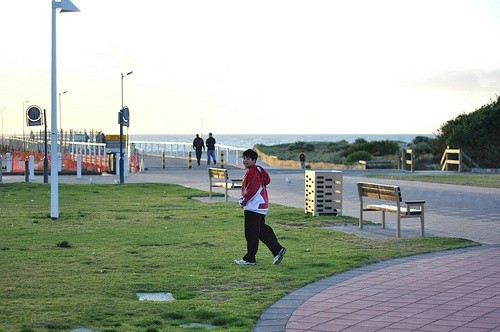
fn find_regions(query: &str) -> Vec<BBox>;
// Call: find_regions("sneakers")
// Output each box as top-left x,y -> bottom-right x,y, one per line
234,259 -> 256,265
273,247 -> 287,265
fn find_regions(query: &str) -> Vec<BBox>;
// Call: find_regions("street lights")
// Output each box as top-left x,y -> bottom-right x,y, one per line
49,1 -> 81,218
119,70 -> 133,183
1,107 -> 7,146
22,100 -> 29,140
59,89 -> 68,143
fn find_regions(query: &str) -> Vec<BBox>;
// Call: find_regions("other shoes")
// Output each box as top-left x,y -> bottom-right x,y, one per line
197,160 -> 200,166
213,160 -> 216,164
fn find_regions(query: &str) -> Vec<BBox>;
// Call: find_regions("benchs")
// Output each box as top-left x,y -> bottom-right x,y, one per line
207,168 -> 243,202
357,182 -> 425,237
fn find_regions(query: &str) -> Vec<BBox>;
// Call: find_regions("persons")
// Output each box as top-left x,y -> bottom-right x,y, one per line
298,150 -> 306,168
85,132 -> 106,149
206,133 -> 216,165
193,134 -> 205,166
234,149 -> 286,265
